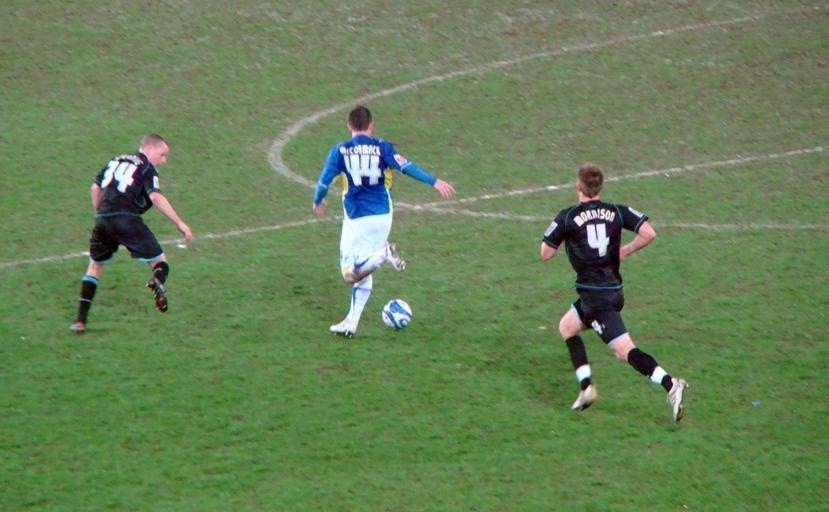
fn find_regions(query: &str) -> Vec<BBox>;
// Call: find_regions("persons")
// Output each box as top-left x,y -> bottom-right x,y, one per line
312,105 -> 457,338
539,162 -> 690,424
67,132 -> 196,334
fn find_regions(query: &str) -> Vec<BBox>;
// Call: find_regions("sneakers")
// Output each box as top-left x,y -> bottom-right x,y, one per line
668,378 -> 689,422
146,277 -> 168,312
330,323 -> 357,338
70,322 -> 85,332
381,243 -> 406,272
571,384 -> 596,412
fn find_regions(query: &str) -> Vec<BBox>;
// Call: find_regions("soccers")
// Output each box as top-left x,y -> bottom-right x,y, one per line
382,299 -> 412,328
